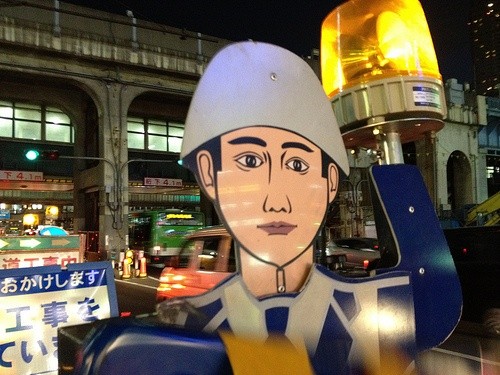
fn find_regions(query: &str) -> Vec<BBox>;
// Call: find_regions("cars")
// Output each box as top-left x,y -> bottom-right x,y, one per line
326,237 -> 381,269
4,221 -> 23,237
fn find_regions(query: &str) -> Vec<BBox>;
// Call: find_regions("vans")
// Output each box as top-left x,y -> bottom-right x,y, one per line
156,223 -> 238,311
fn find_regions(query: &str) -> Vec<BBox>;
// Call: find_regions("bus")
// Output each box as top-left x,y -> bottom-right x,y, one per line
130,208 -> 205,264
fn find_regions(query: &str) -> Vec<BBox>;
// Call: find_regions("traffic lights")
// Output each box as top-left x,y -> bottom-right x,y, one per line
24,148 -> 59,160
176,159 -> 183,167
